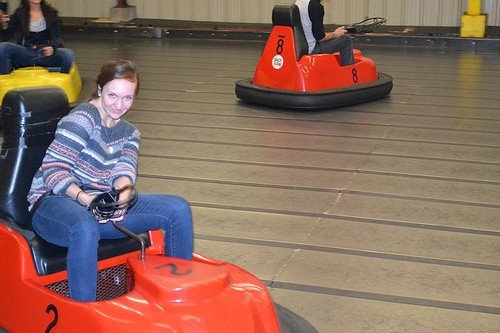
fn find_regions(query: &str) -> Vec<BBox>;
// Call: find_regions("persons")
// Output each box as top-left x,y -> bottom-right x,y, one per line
0,0 -> 75,75
296,0 -> 354,66
27,59 -> 194,301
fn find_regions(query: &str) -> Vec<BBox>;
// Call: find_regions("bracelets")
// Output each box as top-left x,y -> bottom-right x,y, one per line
78,192 -> 87,205
332,32 -> 336,39
75,190 -> 85,202
